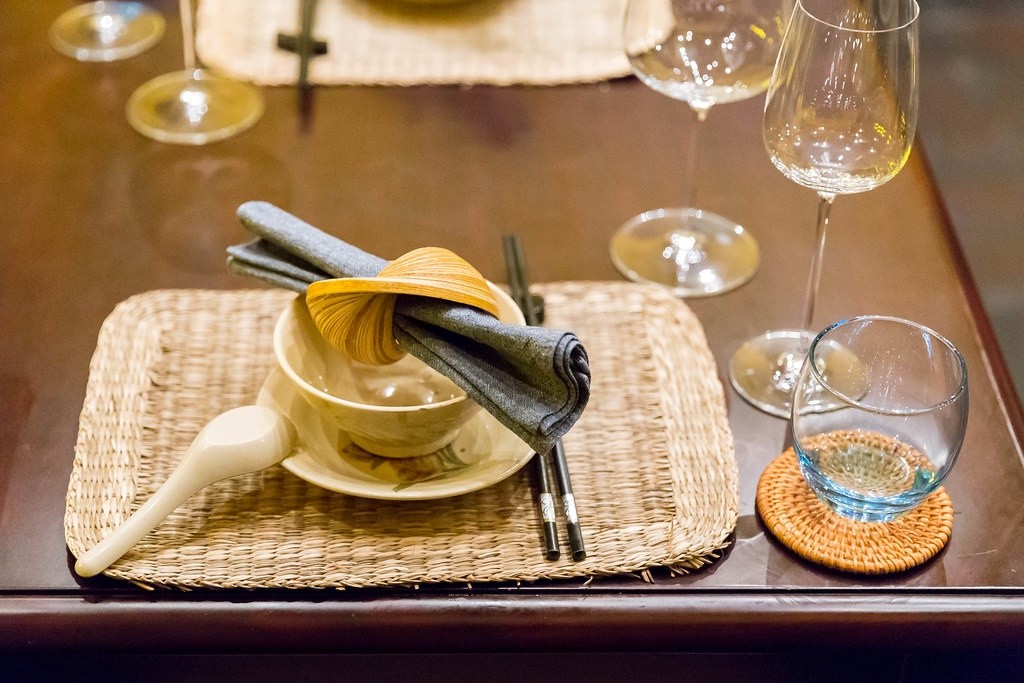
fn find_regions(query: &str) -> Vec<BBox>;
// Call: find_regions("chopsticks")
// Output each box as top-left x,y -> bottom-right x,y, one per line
501,234 -> 586,561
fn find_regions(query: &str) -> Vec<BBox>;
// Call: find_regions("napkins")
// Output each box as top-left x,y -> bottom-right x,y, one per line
224,200 -> 594,458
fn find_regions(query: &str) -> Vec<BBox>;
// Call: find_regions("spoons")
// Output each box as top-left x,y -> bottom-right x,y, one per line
75,406 -> 298,578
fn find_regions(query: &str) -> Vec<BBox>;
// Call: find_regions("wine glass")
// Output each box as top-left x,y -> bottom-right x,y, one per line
123,0 -> 264,145
727,0 -> 918,420
45,0 -> 166,63
607,0 -> 799,297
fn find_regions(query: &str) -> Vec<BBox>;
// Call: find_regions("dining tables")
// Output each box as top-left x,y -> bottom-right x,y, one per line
0,0 -> 1024,683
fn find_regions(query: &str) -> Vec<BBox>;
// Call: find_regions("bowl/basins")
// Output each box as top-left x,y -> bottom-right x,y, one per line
272,279 -> 526,458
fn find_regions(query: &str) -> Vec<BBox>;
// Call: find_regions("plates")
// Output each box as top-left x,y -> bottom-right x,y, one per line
256,360 -> 537,501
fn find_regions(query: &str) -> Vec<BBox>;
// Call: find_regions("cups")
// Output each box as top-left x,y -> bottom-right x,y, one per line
790,317 -> 969,523
